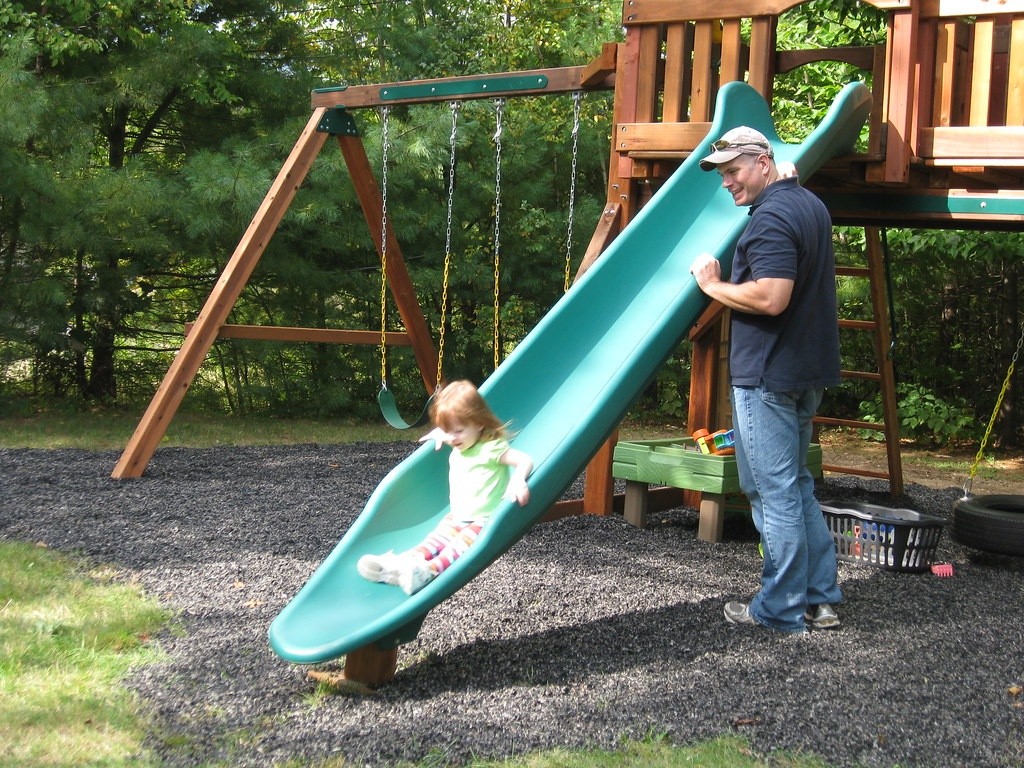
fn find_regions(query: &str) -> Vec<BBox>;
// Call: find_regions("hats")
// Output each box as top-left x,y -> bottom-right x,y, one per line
699,125 -> 775,172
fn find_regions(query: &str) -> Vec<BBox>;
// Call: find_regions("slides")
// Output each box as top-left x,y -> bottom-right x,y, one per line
263,74 -> 881,675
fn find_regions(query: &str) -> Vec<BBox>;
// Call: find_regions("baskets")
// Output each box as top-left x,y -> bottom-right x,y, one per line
820,499 -> 949,572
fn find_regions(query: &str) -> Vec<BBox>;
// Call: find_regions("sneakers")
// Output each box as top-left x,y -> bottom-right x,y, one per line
802,604 -> 842,629
722,602 -> 766,627
356,550 -> 401,586
397,561 -> 433,597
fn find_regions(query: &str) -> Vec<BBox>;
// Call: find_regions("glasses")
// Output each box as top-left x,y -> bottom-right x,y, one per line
708,140 -> 769,151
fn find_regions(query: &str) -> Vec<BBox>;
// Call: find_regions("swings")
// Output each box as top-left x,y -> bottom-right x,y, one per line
376,102 -> 462,430
491,90 -> 580,371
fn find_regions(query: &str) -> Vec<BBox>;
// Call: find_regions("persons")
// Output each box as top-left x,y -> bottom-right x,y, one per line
688,126 -> 842,633
357,381 -> 534,597
